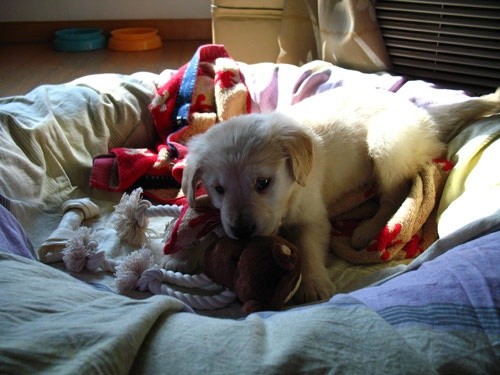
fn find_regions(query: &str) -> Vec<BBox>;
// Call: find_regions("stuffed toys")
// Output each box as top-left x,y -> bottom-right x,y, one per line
62,186 -> 303,323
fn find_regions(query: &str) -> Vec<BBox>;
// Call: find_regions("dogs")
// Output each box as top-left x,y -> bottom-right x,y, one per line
181,84 -> 448,303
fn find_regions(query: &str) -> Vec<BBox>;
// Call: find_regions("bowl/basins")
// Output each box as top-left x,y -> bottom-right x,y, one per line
108,28 -> 163,51
53,27 -> 105,51
